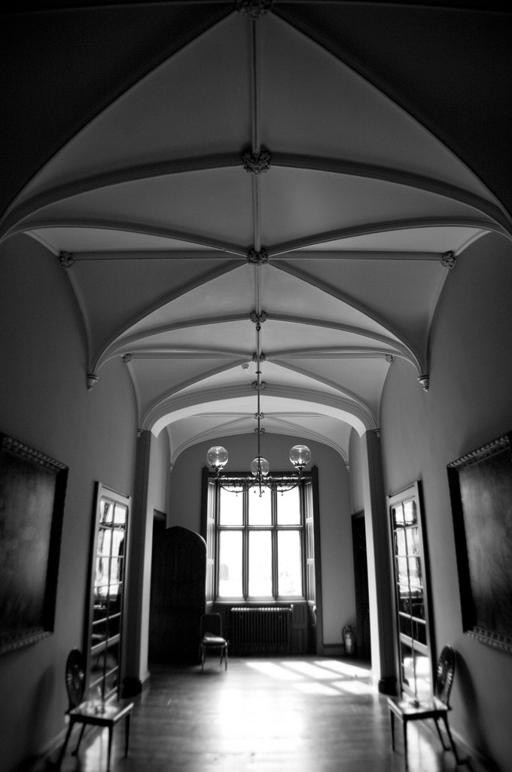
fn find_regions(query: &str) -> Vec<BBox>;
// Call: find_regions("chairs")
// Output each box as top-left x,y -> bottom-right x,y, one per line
200,612 -> 228,672
56,648 -> 135,771
387,645 -> 460,772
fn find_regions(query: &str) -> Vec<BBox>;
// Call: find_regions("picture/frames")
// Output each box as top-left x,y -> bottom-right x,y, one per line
0,430 -> 68,661
445,430 -> 512,653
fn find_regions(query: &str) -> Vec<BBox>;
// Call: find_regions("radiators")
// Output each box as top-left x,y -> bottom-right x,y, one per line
231,608 -> 291,658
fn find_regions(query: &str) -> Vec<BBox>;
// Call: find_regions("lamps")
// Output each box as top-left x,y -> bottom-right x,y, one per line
206,392 -> 312,497
